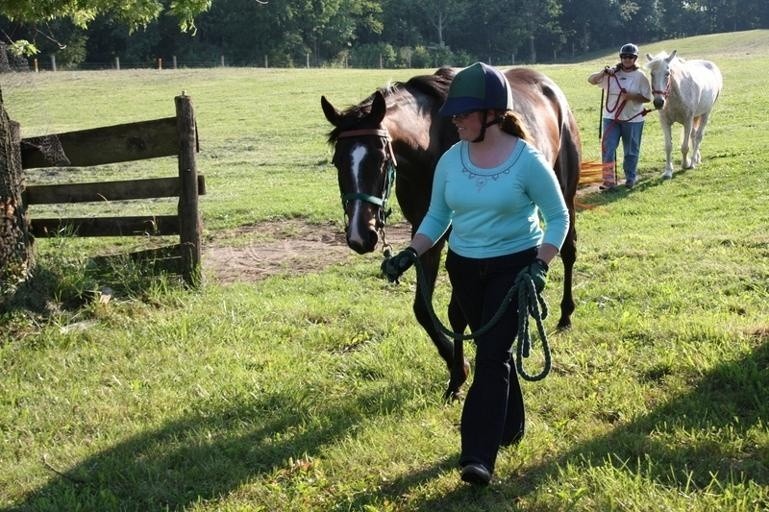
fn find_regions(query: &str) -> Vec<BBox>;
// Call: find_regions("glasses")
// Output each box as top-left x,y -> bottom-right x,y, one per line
621,54 -> 636,60
449,111 -> 478,119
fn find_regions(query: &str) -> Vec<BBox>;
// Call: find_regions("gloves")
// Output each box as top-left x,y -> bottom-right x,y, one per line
516,258 -> 549,296
380,246 -> 418,283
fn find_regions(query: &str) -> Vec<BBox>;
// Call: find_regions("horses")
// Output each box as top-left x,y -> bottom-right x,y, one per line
645,48 -> 724,180
320,65 -> 583,401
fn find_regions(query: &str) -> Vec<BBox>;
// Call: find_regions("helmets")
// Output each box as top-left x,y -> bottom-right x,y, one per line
437,61 -> 514,114
619,43 -> 638,56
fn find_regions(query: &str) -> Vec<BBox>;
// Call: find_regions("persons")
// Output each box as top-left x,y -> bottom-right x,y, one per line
380,60 -> 570,485
587,42 -> 652,191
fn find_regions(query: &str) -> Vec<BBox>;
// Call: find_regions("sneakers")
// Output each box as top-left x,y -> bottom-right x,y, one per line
460,462 -> 491,485
599,180 -> 617,190
625,179 -> 634,189
